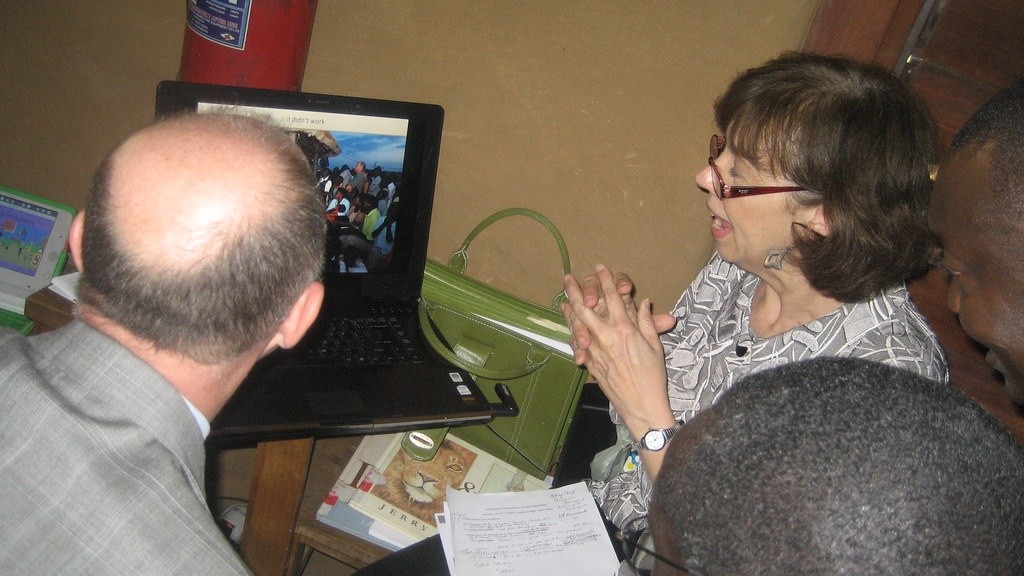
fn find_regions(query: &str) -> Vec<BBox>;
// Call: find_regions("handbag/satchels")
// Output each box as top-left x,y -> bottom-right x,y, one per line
401,208 -> 589,480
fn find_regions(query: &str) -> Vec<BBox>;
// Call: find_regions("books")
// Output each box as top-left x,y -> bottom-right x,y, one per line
316,430 -> 554,551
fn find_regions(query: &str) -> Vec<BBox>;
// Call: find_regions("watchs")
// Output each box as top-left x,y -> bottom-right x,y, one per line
631,423 -> 680,452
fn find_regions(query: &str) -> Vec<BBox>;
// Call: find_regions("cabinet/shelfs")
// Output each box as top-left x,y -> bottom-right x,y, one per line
24,283 -> 394,576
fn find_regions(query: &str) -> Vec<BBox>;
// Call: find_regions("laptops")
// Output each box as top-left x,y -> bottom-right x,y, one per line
0,185 -> 78,337
150,80 -> 495,444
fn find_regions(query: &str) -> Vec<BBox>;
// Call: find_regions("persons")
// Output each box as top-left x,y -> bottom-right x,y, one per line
0,112 -> 325,576
560,53 -> 951,556
650,357 -> 1024,576
317,161 -> 401,273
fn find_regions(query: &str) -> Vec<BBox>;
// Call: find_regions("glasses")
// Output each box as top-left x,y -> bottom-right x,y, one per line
708,133 -> 806,200
615,514 -> 698,576
